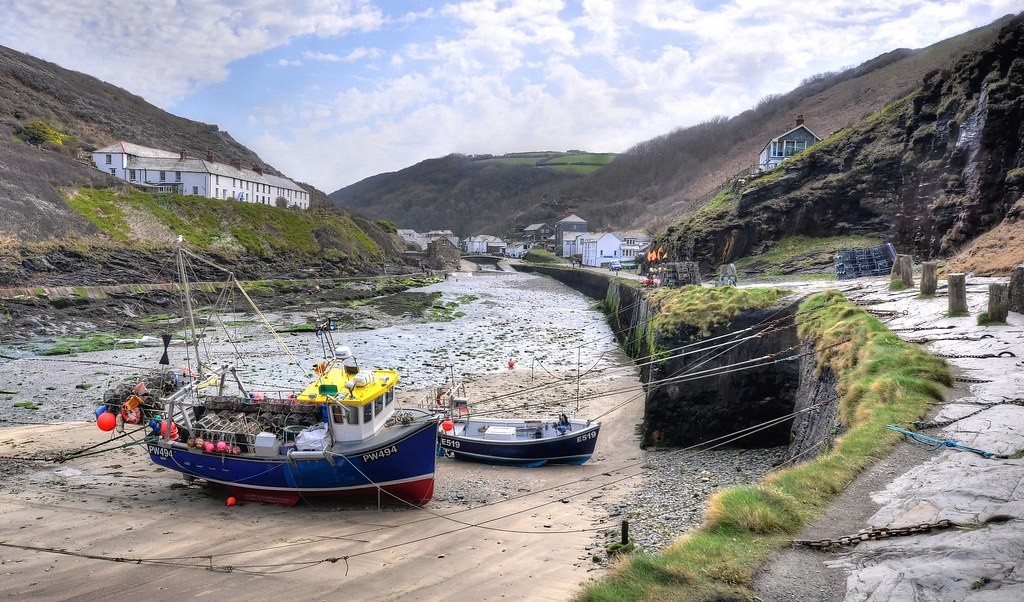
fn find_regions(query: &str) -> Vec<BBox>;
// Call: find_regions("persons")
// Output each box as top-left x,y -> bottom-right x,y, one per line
649,266 -> 666,279
572,259 -> 582,268
422,264 -> 447,281
557,414 -> 572,435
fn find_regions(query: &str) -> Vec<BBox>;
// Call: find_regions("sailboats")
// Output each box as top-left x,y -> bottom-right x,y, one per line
148,248 -> 440,508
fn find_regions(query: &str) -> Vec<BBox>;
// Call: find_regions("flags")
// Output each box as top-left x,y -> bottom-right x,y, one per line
647,246 -> 668,261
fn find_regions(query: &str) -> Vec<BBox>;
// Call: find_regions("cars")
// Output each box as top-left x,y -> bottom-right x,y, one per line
608,260 -> 638,272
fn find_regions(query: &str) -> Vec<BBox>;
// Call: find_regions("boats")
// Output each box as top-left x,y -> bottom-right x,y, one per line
436,416 -> 601,467
